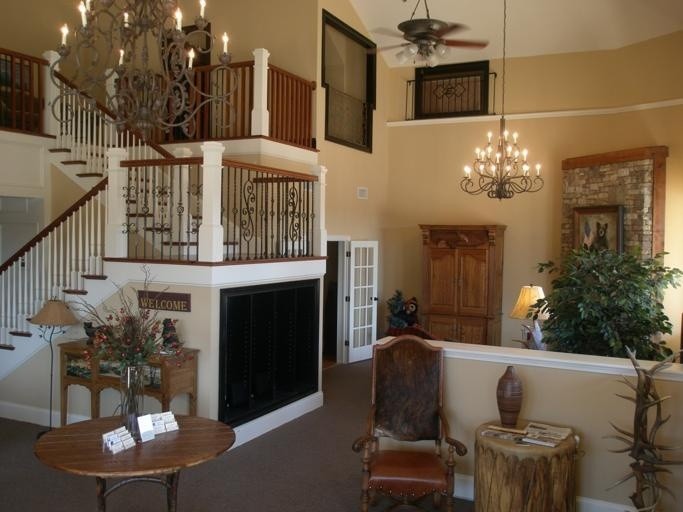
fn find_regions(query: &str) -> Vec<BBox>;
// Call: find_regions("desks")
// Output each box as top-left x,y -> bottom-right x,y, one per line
33,416 -> 236,512
58,338 -> 199,425
474,418 -> 577,512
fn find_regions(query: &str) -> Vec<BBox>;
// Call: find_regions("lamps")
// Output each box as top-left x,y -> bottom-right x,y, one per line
510,284 -> 549,349
50,0 -> 237,142
460,0 -> 544,199
396,39 -> 450,67
30,301 -> 79,440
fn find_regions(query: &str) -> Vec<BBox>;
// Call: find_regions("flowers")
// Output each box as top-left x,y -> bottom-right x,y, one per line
71,264 -> 194,417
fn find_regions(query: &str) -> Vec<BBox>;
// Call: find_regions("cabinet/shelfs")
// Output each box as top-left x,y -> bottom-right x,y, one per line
418,224 -> 507,346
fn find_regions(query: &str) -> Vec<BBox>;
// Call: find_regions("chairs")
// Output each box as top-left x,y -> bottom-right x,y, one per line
353,335 -> 468,512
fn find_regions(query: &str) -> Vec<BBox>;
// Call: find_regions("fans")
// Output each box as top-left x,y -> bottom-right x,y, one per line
365,0 -> 488,54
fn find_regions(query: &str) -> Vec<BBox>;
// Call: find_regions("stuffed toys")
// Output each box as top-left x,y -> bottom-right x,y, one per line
394,295 -> 419,326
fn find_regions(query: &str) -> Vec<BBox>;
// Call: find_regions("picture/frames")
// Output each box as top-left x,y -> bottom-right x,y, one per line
573,204 -> 623,256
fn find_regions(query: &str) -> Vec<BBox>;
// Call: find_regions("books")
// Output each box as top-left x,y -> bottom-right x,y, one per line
479,420 -> 573,448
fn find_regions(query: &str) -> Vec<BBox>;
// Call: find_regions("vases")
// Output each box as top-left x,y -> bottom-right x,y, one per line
119,366 -> 143,441
497,366 -> 523,426
